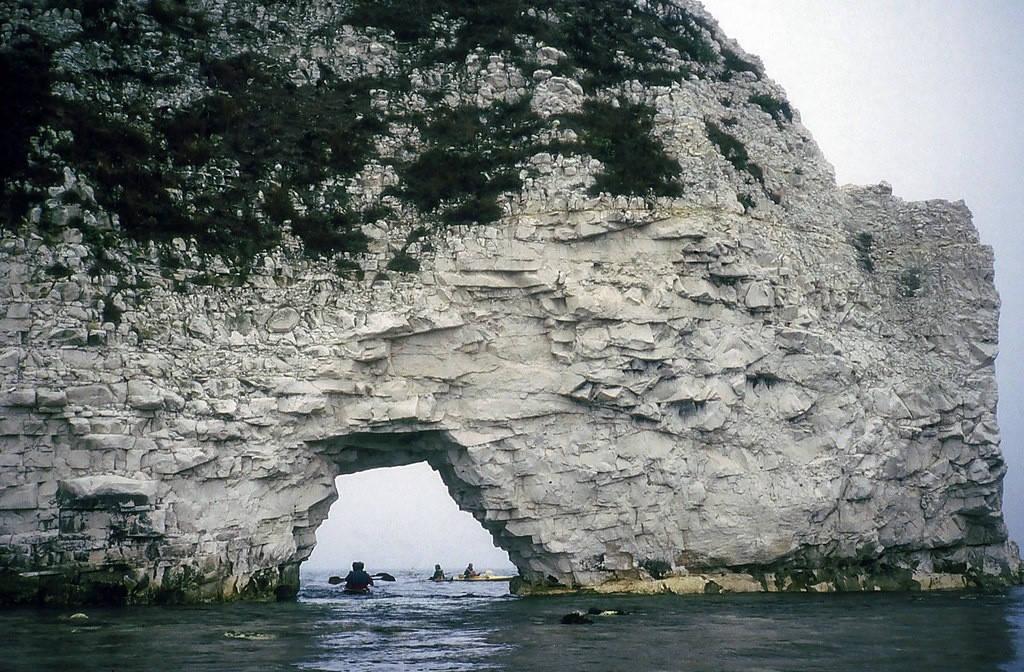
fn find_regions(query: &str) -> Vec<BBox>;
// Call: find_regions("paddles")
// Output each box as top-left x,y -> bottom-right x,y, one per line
329,572 -> 389,585
416,571 -> 451,582
459,569 -> 493,579
329,575 -> 396,585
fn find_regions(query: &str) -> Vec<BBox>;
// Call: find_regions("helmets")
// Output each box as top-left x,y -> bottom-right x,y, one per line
352,562 -> 364,571
469,562 -> 473,568
435,564 -> 442,570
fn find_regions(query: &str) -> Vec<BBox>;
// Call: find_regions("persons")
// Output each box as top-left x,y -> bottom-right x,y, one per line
433,564 -> 445,579
464,563 -> 477,575
345,562 -> 374,589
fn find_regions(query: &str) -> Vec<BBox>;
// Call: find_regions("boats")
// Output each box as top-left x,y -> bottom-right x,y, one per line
342,589 -> 366,596
431,574 -> 515,581
